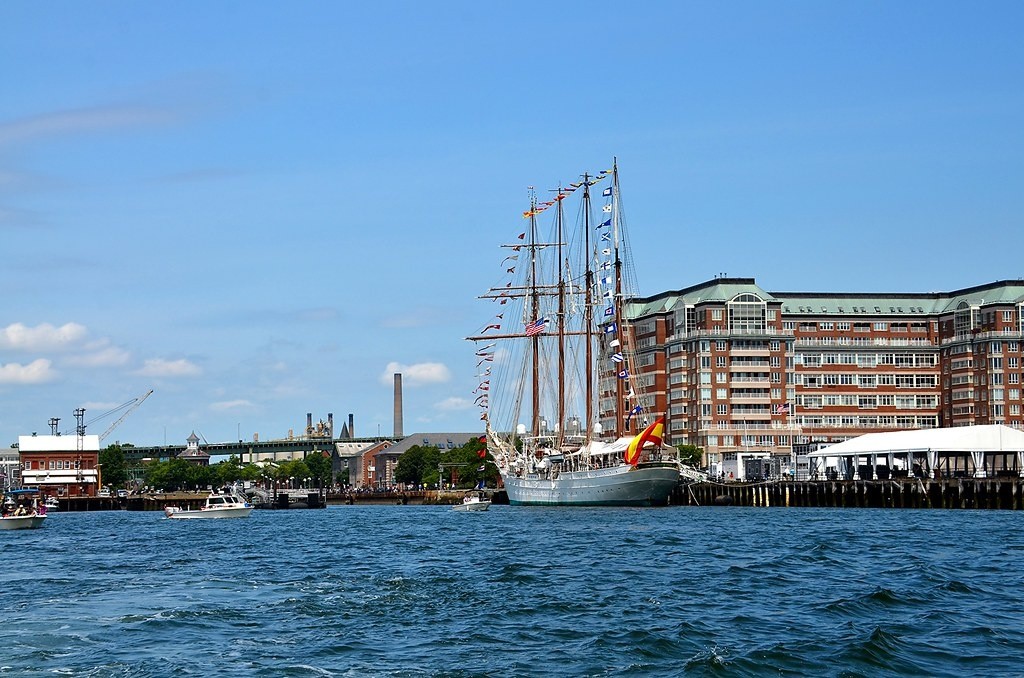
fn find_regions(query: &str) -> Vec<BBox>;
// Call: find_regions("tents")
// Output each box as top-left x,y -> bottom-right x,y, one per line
566,435 -> 676,472
805,424 -> 1024,480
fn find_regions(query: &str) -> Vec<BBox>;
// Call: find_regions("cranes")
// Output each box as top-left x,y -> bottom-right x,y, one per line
46,389 -> 155,498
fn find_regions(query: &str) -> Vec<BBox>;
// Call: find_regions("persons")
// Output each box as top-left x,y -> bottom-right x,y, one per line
851,466 -> 855,480
718,471 -> 733,483
37,501 -> 48,515
764,471 -> 768,480
323,482 -> 456,493
233,479 -> 241,486
784,468 -> 795,481
464,493 -> 474,502
590,455 -> 601,465
28,507 -> 37,516
814,467 -> 819,480
13,504 -> 27,516
0,499 -> 7,517
402,493 -> 408,505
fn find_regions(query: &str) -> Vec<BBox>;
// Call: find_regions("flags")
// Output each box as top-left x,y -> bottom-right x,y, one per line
477,447 -> 486,458
474,479 -> 485,490
777,403 -> 789,413
476,464 -> 485,471
480,437 -> 487,443
625,413 -> 667,469
599,187 -> 613,300
604,307 -> 653,430
525,317 -> 545,336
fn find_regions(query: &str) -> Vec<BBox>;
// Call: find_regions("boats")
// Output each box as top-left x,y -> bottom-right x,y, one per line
452,489 -> 493,512
0,489 -> 48,530
163,493 -> 255,519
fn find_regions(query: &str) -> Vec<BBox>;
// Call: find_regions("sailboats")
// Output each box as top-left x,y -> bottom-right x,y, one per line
465,155 -> 682,508
